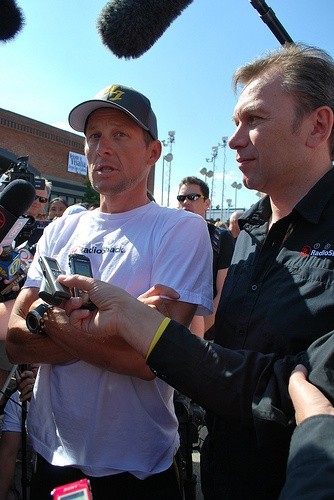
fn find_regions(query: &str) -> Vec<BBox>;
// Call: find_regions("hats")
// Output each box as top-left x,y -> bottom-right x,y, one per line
68,83 -> 158,140
61,205 -> 87,217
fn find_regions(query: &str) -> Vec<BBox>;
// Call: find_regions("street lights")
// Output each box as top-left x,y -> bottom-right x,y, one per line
161,153 -> 173,206
231,181 -> 242,211
200,167 -> 213,185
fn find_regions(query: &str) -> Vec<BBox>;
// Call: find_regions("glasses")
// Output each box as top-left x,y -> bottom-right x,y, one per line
177,193 -> 207,203
34,195 -> 48,203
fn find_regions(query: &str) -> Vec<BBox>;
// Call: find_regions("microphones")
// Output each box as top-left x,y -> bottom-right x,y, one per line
18,364 -> 31,382
0,180 -> 46,280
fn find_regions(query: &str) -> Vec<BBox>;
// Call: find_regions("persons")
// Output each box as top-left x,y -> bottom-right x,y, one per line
205,209 -> 245,246
200,45 -> 334,499
177,176 -> 236,344
0,363 -> 37,500
0,159 -> 98,404
6,85 -> 216,500
59,274 -> 334,500
